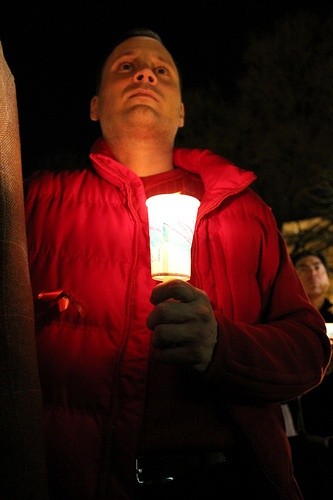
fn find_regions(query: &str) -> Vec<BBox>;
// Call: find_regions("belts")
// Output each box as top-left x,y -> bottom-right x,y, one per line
136,452 -> 229,485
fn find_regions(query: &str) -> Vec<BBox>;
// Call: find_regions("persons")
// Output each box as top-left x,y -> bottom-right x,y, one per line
288,250 -> 333,436
24,29 -> 332,500
